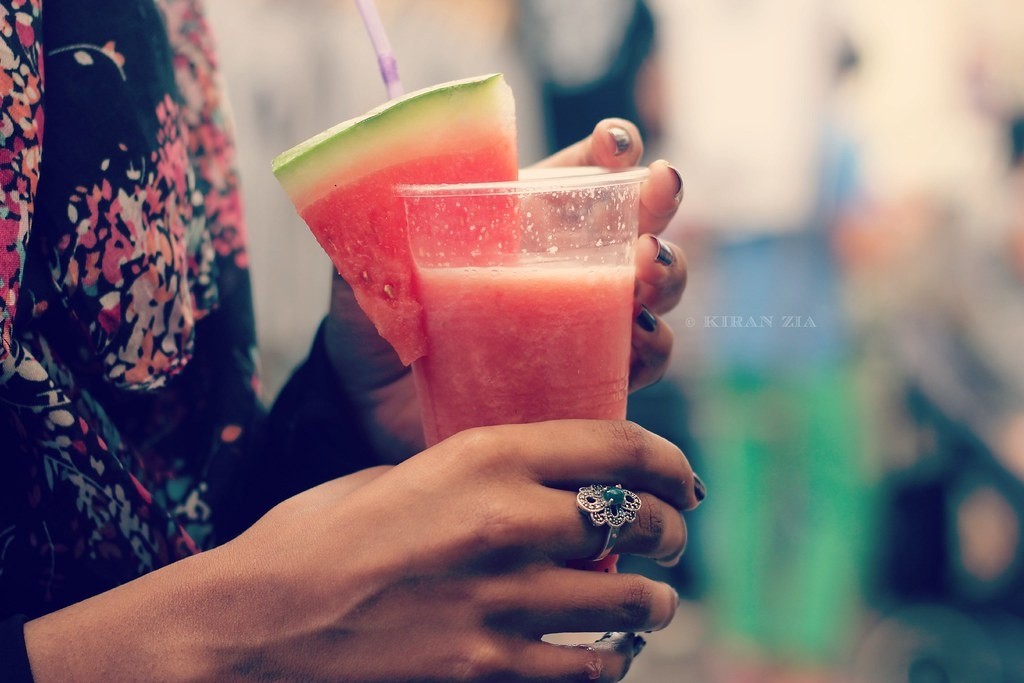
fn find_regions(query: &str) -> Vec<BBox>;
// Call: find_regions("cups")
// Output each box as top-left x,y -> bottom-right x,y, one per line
387,166 -> 651,574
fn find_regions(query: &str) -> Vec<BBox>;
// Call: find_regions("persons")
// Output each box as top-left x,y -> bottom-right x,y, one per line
873,113 -> 1024,609
667,35 -> 934,683
505,2 -> 707,603
0,0 -> 705,683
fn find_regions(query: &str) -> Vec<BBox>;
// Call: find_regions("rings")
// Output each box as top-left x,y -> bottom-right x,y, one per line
577,484 -> 642,561
573,642 -> 603,680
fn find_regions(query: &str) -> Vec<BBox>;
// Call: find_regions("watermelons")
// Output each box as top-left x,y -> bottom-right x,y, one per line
272,71 -> 522,366
